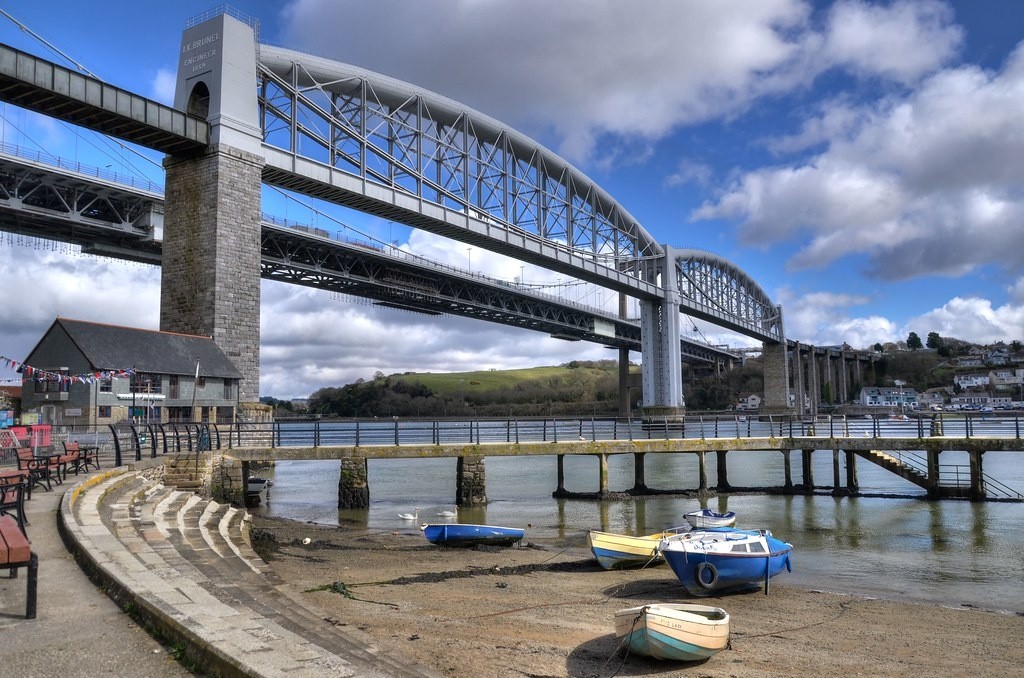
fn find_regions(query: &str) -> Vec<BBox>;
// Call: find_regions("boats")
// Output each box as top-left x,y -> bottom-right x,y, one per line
683,508 -> 736,529
886,414 -> 909,422
859,414 -> 873,420
421,522 -> 524,546
739,416 -> 747,422
585,529 -> 679,570
614,603 -> 733,663
658,509 -> 796,598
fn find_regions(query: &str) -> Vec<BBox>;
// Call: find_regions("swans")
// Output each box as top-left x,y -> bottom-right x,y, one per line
433,505 -> 459,517
397,507 -> 420,520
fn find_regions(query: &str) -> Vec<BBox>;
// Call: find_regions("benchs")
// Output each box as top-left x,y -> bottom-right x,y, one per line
0,470 -> 27,527
61,440 -> 100,474
44,455 -> 79,480
0,483 -> 39,620
11,446 -> 62,492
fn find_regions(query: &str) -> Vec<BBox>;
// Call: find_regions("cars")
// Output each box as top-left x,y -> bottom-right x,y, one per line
905,400 -> 1024,415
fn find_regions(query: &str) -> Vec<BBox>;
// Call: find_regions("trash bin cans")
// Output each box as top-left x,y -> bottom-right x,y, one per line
139,433 -> 147,443
197,432 -> 210,450
